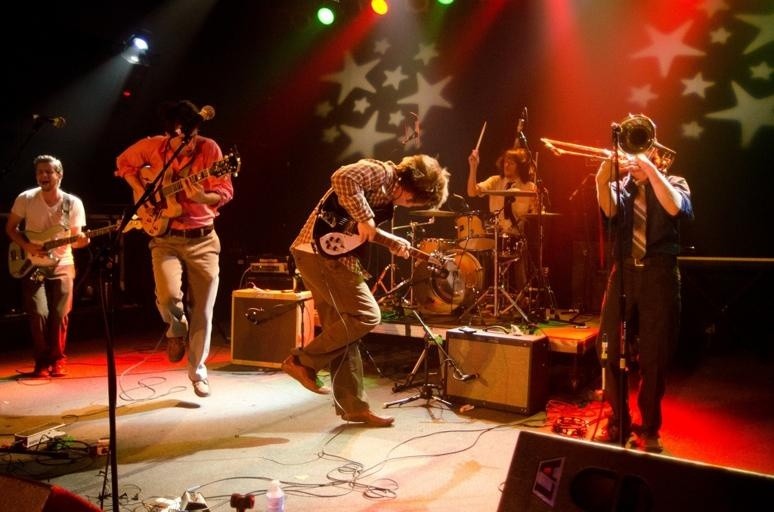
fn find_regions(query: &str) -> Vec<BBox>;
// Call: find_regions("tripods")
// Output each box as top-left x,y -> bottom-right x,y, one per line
371,209 -> 560,390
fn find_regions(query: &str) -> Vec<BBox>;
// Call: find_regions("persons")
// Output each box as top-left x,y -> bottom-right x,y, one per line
281,154 -> 452,427
467,148 -> 539,290
116,99 -> 234,397
590,153 -> 697,452
6,154 -> 92,377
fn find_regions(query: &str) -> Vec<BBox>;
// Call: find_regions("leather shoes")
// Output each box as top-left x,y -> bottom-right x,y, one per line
281,353 -> 331,394
343,408 -> 394,425
594,425 -> 630,445
645,436 -> 662,451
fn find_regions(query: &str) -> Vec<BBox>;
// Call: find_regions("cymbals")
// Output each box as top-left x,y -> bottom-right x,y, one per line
481,190 -> 537,195
409,209 -> 456,217
521,212 -> 561,216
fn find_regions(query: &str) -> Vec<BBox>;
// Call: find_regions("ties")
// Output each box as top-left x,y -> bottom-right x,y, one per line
504,182 -> 514,219
632,183 -> 647,261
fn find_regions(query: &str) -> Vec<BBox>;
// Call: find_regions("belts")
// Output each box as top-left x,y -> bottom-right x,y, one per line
625,256 -> 662,266
167,225 -> 214,238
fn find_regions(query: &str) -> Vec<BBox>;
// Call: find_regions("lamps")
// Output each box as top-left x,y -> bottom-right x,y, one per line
130,30 -> 153,52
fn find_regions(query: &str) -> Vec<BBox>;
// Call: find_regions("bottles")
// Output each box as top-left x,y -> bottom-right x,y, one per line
267,480 -> 283,511
292,273 -> 301,293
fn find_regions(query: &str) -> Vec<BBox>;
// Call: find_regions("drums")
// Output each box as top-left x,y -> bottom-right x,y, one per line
413,237 -> 456,269
498,233 -> 521,258
411,248 -> 484,317
454,211 -> 495,251
494,233 -> 526,262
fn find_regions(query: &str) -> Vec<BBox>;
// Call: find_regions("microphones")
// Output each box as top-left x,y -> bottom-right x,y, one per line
610,122 -> 623,135
513,118 -> 525,148
179,104 -> 216,133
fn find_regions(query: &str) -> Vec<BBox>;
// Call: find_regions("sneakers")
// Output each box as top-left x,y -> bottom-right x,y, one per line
168,337 -> 185,362
36,360 -> 65,377
190,374 -> 209,397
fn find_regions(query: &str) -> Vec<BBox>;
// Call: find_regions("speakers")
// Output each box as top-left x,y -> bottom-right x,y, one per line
497,430 -> 774,511
230,287 -> 315,368
443,326 -> 550,416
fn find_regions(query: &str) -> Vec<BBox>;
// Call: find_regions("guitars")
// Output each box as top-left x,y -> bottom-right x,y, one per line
8,219 -> 142,279
313,191 -> 458,279
133,146 -> 241,237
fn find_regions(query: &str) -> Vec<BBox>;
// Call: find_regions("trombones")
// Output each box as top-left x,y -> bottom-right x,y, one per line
540,114 -> 677,173
